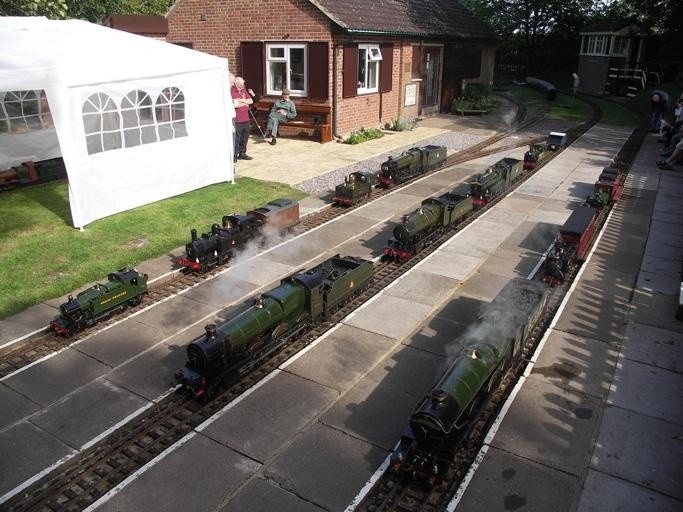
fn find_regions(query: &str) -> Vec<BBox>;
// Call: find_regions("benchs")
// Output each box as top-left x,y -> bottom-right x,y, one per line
251,101 -> 332,144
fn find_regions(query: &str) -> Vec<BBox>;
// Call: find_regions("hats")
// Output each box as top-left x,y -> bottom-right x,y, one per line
282,89 -> 292,95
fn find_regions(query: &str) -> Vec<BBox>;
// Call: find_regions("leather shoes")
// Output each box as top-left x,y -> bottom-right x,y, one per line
260,132 -> 270,139
272,139 -> 277,145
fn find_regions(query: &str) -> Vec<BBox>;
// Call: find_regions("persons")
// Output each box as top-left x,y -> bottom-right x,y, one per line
232,77 -> 256,163
259,88 -> 297,145
648,90 -> 683,170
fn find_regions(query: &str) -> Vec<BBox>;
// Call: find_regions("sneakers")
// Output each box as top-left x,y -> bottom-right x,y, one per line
234,151 -> 252,164
